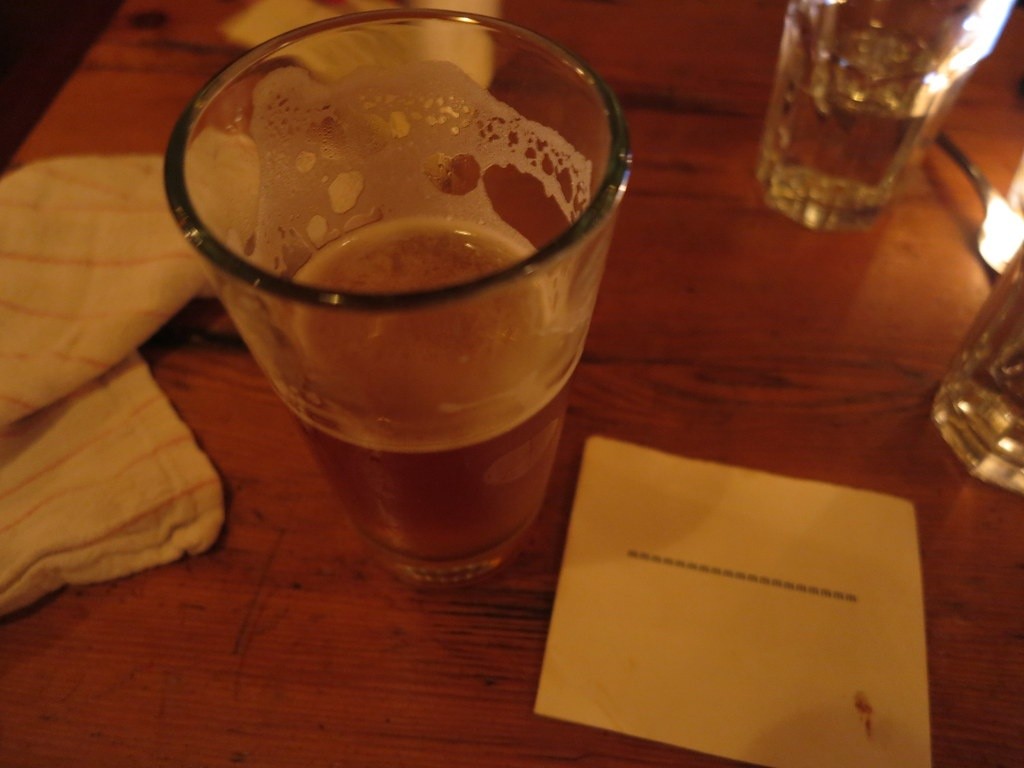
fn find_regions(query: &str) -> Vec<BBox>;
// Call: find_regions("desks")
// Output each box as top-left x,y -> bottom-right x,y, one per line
1,0 -> 1024,768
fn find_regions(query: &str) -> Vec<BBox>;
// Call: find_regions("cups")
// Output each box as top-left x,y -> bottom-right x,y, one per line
753,0 -> 1017,233
162,9 -> 631,586
932,240 -> 1024,494
